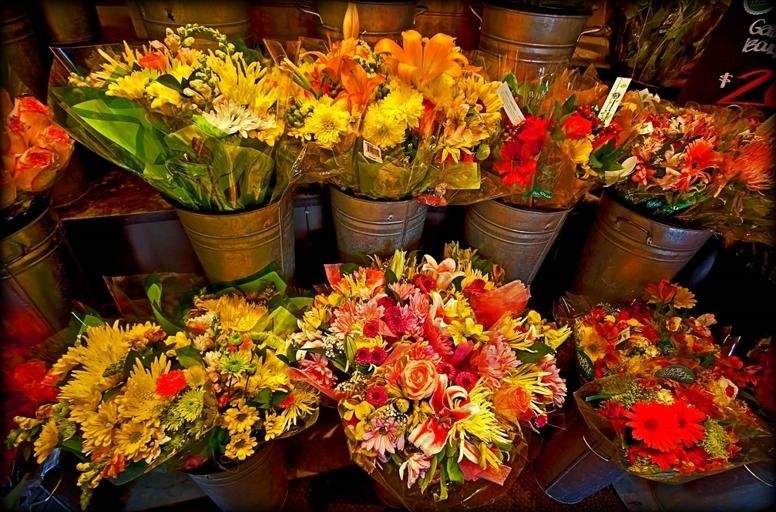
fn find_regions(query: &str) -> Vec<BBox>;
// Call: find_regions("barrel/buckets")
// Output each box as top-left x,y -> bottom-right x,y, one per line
0,1 -> 776,512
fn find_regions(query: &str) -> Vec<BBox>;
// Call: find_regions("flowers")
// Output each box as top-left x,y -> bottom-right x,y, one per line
1,1 -> 775,512
